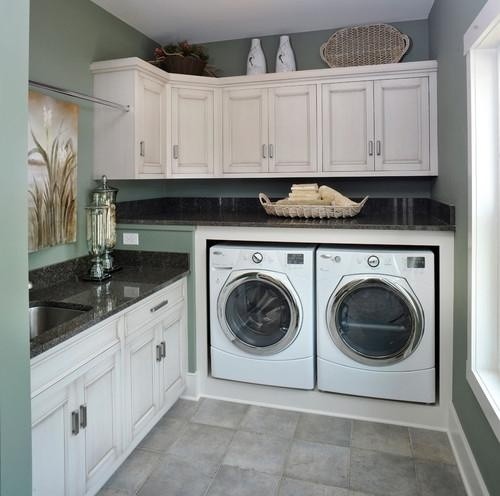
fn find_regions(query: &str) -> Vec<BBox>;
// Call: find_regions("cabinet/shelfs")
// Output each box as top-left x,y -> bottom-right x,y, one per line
88,57 -> 220,182
222,60 -> 438,178
31,275 -> 187,496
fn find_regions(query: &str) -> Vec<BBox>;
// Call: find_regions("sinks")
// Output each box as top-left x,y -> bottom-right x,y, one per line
30,299 -> 93,340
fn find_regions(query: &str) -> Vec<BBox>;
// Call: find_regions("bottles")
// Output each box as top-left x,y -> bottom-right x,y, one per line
245,37 -> 267,76
274,36 -> 297,73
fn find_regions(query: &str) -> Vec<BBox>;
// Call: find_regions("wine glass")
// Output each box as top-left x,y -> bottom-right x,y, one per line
85,195 -> 108,281
90,175 -> 121,272
91,282 -> 115,319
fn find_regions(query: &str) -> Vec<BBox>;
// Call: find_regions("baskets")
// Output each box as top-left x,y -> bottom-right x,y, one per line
258,192 -> 369,219
161,56 -> 208,76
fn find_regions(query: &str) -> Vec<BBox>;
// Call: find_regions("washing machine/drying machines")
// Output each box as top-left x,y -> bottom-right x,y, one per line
209,245 -> 314,391
314,244 -> 437,404
